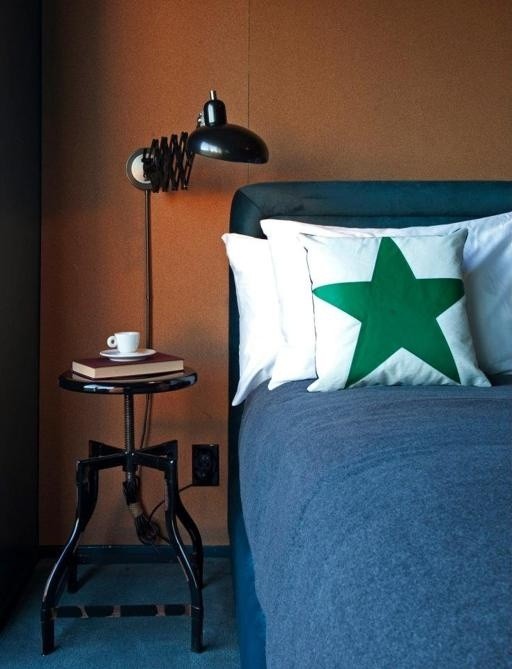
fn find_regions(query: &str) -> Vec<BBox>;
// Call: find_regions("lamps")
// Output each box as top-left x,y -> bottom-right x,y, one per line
130,91 -> 270,193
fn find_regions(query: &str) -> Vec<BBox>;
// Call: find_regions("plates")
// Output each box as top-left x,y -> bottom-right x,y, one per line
100,348 -> 157,362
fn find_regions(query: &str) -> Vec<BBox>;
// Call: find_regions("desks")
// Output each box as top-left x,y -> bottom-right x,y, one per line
40,365 -> 205,657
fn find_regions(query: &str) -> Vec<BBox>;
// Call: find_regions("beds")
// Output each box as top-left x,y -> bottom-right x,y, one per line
228,179 -> 511,669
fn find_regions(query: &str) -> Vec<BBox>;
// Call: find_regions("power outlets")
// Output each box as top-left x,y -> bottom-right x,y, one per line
191,442 -> 220,488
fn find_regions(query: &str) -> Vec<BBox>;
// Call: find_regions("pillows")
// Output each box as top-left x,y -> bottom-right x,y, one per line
260,212 -> 512,390
220,231 -> 281,407
296,227 -> 494,392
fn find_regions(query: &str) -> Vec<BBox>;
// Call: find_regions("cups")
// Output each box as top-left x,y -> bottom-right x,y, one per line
107,332 -> 140,354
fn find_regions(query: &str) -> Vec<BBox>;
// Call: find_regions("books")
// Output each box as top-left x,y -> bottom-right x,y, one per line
73,349 -> 185,381
72,371 -> 184,385
